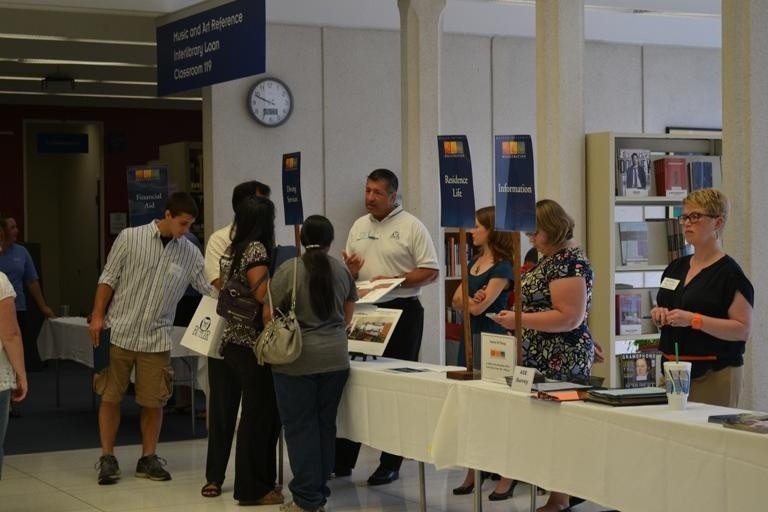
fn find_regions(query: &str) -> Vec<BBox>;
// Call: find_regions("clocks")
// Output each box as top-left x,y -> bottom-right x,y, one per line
247,76 -> 294,127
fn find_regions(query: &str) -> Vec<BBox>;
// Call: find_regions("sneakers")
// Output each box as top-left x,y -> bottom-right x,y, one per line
279,499 -> 325,512
95,454 -> 123,484
134,453 -> 172,482
236,485 -> 284,505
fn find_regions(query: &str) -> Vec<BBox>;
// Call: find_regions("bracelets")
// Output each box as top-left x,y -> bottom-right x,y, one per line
396,274 -> 409,288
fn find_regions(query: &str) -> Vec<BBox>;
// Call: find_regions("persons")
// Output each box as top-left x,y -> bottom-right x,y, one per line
216,194 -> 289,506
262,213 -> 359,511
84,192 -> 220,486
451,205 -> 518,501
1,267 -> 28,494
331,167 -> 441,485
1,215 -> 56,372
654,187 -> 755,412
201,179 -> 274,498
491,199 -> 596,511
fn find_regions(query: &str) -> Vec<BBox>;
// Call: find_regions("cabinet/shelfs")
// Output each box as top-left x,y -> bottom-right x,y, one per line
444,231 -> 475,368
585,130 -> 725,390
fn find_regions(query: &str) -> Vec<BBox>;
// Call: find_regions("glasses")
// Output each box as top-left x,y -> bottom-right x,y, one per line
678,213 -> 715,223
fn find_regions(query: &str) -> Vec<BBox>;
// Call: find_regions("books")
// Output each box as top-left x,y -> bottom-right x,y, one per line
707,410 -> 768,423
531,381 -> 590,393
587,386 -> 668,405
723,418 -> 768,434
615,147 -> 714,387
447,235 -> 475,366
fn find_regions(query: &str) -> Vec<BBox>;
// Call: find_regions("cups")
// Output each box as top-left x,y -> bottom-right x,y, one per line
60,305 -> 69,318
663,360 -> 692,409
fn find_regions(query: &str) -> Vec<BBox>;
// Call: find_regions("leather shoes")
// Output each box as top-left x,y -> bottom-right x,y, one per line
367,461 -> 400,485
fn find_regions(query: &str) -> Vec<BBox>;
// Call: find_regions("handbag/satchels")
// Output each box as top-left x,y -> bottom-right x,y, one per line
216,239 -> 267,330
179,294 -> 226,361
252,257 -> 302,365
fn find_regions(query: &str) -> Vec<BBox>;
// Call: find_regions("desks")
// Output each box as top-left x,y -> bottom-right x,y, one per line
35,316 -> 198,432
277,358 -> 478,511
439,366 -> 768,512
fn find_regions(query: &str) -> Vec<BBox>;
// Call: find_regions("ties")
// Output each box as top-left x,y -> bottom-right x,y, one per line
633,167 -> 638,188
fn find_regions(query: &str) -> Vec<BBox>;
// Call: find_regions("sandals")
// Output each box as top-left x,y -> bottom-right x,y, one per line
201,480 -> 221,498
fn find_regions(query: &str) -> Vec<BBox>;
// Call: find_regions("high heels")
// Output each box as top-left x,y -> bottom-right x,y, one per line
453,472 -> 485,495
488,478 -> 518,501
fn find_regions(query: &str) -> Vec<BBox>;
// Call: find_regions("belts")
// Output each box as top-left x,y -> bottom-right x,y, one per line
378,295 -> 418,308
